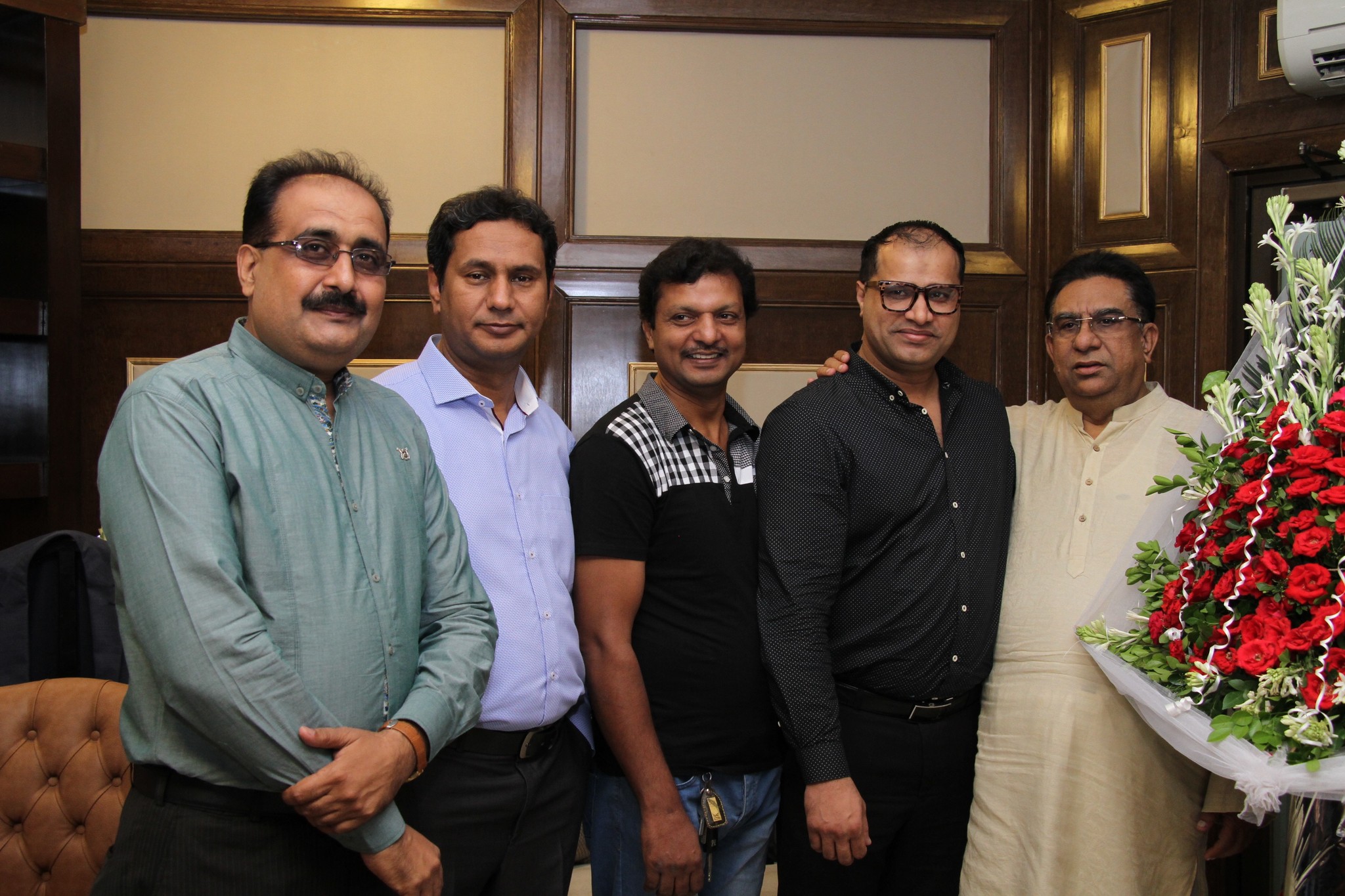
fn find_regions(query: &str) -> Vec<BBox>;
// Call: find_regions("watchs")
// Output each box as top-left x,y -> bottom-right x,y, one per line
379,721 -> 427,783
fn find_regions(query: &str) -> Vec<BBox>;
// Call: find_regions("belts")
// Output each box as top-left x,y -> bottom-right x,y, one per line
460,722 -> 573,760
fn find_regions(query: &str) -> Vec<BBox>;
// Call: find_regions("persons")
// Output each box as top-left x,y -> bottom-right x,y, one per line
369,181 -> 620,896
569,240 -> 795,895
750,219 -> 1015,896
94,147 -> 499,895
815,250 -> 1257,896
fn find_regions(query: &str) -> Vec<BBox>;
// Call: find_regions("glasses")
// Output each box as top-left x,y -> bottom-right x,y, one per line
254,236 -> 395,275
864,281 -> 964,315
1045,311 -> 1151,338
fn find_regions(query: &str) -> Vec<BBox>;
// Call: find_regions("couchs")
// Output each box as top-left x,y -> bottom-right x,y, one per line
0,678 -> 132,896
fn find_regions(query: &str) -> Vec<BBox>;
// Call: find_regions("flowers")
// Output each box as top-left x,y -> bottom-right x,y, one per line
1075,139 -> 1345,773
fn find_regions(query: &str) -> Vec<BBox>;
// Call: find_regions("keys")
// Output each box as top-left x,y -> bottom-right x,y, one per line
697,789 -> 719,882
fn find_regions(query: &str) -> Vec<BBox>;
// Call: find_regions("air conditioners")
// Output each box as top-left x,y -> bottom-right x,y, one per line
1277,0 -> 1345,97
